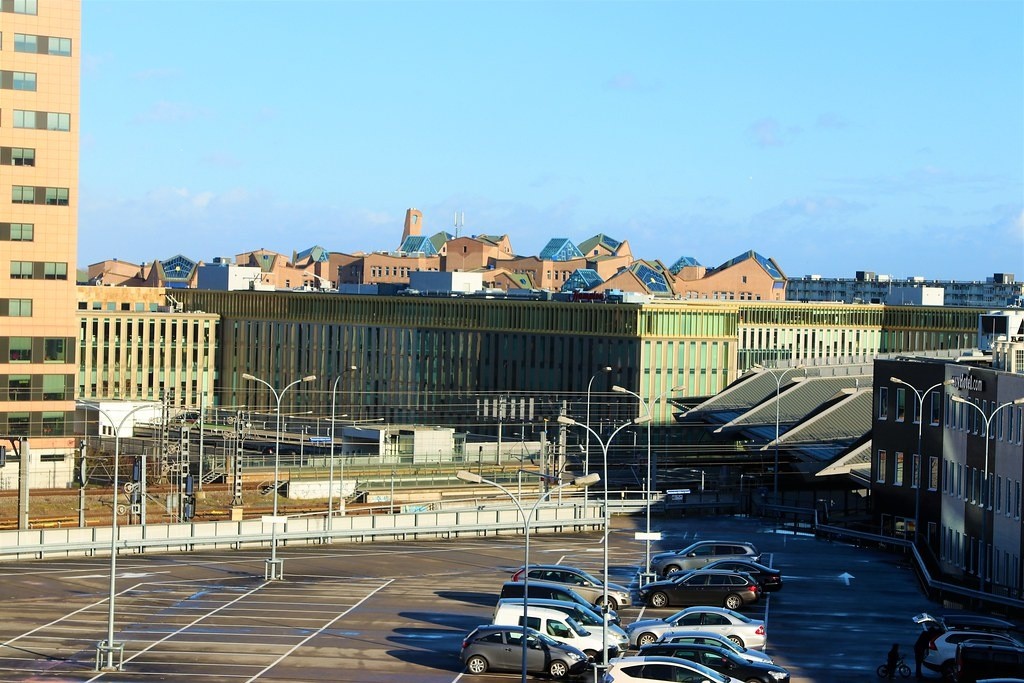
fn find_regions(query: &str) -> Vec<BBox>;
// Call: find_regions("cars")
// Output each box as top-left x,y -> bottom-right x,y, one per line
913,613 -> 1024,683
603,606 -> 789,683
639,541 -> 781,611
459,624 -> 588,680
262,444 -> 299,454
510,564 -> 633,611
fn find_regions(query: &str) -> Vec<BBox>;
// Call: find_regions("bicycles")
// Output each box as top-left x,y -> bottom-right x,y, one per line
877,655 -> 912,679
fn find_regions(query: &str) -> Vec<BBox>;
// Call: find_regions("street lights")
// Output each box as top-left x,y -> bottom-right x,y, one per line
612,385 -> 685,586
243,373 -> 318,581
950,396 -> 1024,596
456,470 -> 601,683
890,377 -> 955,549
556,415 -> 654,671
584,366 -> 613,533
753,363 -> 804,534
75,403 -> 165,669
328,366 -> 358,544
220,408 -> 385,441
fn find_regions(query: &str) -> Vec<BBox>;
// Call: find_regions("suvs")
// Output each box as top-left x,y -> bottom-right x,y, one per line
490,583 -> 632,662
170,412 -> 206,424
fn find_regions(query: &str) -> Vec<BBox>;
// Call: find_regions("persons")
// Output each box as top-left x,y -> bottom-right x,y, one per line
914,627 -> 936,680
888,644 -> 899,680
